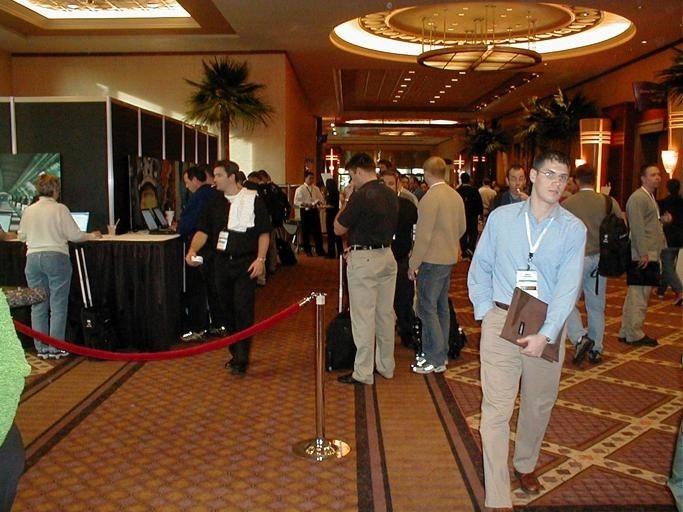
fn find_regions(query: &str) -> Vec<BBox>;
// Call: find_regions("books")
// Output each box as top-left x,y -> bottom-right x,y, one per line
497,286 -> 565,364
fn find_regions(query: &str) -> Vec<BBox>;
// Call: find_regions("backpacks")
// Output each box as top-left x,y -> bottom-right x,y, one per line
598,194 -> 632,278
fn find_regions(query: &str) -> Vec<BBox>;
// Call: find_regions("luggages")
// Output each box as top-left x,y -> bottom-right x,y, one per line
75,243 -> 117,360
325,252 -> 357,371
275,226 -> 297,266
412,280 -> 468,360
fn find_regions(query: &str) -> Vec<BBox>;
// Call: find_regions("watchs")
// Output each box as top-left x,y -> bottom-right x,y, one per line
543,335 -> 552,344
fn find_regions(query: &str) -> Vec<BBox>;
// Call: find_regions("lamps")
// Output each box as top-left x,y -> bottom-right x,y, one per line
661,150 -> 676,180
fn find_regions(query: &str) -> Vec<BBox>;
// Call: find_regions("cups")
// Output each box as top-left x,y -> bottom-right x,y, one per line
107,224 -> 116,235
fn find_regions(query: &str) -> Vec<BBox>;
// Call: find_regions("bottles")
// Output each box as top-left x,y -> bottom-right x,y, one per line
602,234 -> 609,245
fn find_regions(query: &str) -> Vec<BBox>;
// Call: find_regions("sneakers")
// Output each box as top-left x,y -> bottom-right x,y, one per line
410,357 -> 448,374
180,331 -> 208,343
572,335 -> 602,367
37,350 -> 70,360
208,323 -> 229,338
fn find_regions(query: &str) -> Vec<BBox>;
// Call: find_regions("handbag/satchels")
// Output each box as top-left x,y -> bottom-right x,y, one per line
625,258 -> 661,287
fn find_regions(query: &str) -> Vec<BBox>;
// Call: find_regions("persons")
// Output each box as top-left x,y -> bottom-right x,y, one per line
294,172 -> 344,259
456,164 -> 528,263
170,158 -> 297,376
462,149 -> 588,512
333,152 -> 466,384
2,171 -> 103,360
559,164 -> 627,366
0,287 -> 29,512
619,163 -> 682,346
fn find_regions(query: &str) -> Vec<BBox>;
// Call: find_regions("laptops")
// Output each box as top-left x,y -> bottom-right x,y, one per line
1,212 -> 12,231
153,207 -> 171,228
70,212 -> 90,231
141,208 -> 175,234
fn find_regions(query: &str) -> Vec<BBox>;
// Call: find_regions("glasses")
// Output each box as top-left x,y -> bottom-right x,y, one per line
532,166 -> 570,185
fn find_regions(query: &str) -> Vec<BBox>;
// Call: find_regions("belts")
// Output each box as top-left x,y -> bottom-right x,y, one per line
495,302 -> 509,310
219,252 -> 253,260
351,244 -> 389,250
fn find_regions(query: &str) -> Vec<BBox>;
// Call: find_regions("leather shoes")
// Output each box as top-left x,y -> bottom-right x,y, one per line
226,353 -> 246,375
515,471 -> 540,494
338,373 -> 361,384
618,336 -> 658,347
